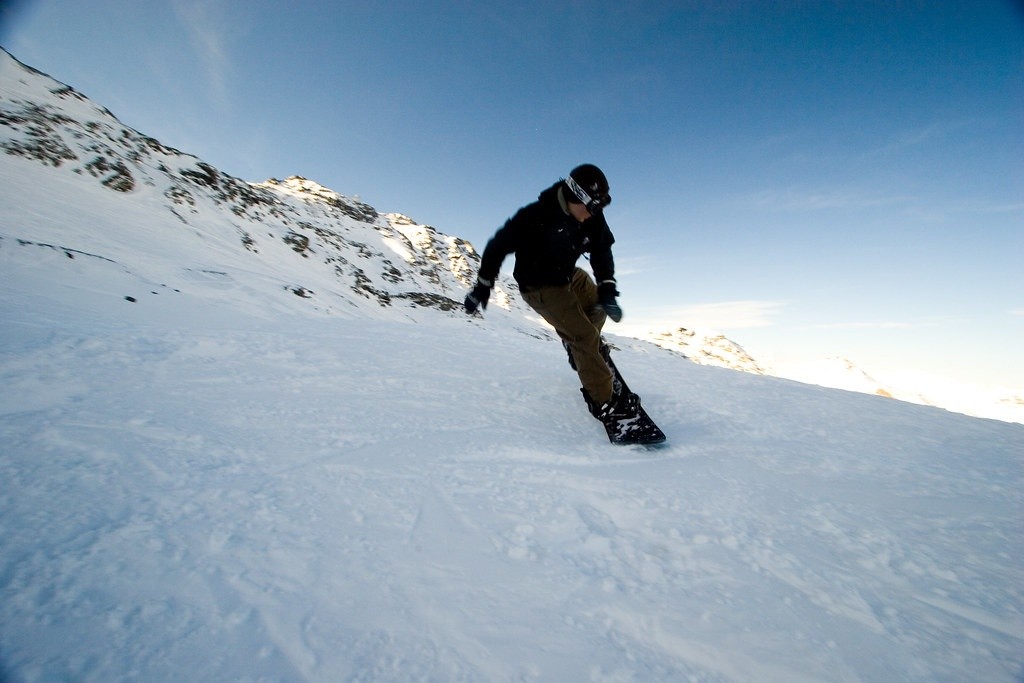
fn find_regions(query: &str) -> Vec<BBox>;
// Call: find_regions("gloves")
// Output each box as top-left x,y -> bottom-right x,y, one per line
464,276 -> 492,312
596,279 -> 622,322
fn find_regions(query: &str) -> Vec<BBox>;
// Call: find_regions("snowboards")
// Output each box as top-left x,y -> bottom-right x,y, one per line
561,334 -> 667,446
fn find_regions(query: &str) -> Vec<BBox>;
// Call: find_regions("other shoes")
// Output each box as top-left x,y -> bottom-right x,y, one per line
590,393 -> 641,421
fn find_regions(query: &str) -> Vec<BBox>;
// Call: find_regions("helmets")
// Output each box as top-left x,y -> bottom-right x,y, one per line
562,164 -> 612,216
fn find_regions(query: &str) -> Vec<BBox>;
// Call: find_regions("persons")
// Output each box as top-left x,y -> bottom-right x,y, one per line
465,164 -> 646,420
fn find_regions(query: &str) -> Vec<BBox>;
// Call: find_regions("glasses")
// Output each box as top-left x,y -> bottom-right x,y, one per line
566,177 -> 611,216
